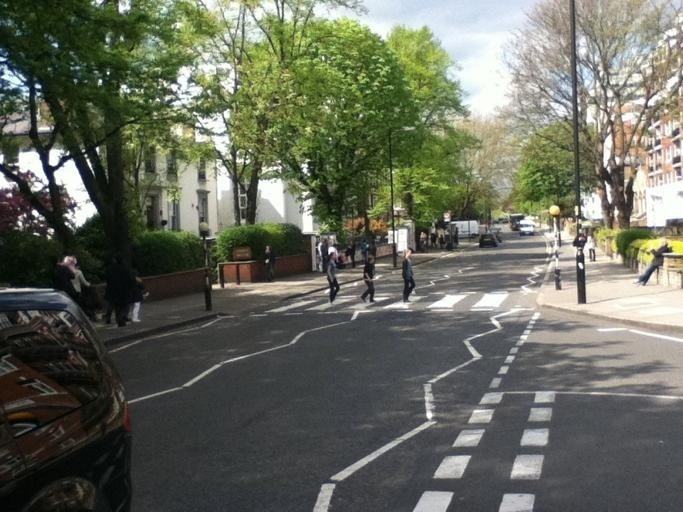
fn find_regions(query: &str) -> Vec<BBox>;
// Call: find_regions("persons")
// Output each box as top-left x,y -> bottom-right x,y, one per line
360,255 -> 375,304
316,237 -> 355,274
633,237 -> 672,288
430,232 -> 435,248
401,248 -> 415,302
262,245 -> 272,281
439,234 -> 443,249
326,250 -> 340,306
51,253 -> 151,330
587,233 -> 595,262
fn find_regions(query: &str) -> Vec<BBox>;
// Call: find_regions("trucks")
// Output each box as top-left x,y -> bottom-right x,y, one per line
508,213 -> 525,231
449,219 -> 479,239
519,219 -> 535,236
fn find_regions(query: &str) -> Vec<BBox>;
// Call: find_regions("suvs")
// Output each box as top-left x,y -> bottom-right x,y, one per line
0,279 -> 137,512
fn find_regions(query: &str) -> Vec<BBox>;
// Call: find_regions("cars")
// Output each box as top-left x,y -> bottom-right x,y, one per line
490,227 -> 504,242
479,233 -> 499,248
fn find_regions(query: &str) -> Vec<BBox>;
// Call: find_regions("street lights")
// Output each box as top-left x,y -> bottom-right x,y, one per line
197,217 -> 213,312
549,205 -> 563,290
389,125 -> 417,268
